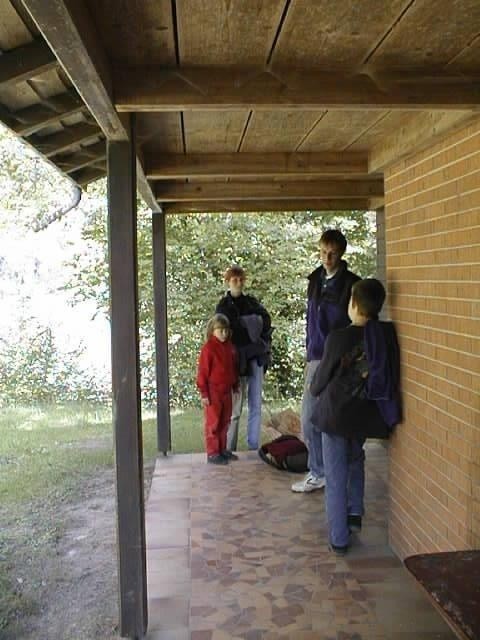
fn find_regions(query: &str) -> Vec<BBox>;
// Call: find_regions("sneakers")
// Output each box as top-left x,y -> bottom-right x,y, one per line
348,513 -> 362,535
328,542 -> 345,557
291,474 -> 326,493
206,456 -> 228,464
222,452 -> 238,460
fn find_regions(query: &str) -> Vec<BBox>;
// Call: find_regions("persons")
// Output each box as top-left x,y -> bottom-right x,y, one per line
195,312 -> 239,467
309,278 -> 400,555
213,266 -> 272,452
289,230 -> 362,493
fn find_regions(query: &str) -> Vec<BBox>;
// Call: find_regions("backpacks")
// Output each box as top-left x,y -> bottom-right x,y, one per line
258,435 -> 309,472
